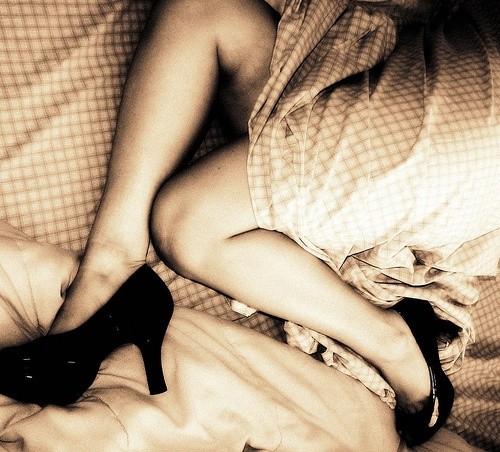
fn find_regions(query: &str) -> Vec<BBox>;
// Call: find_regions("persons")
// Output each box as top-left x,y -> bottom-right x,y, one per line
0,0 -> 500,451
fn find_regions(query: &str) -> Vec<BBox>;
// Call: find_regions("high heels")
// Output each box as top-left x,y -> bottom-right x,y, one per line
0,264 -> 174,406
382,297 -> 457,448
